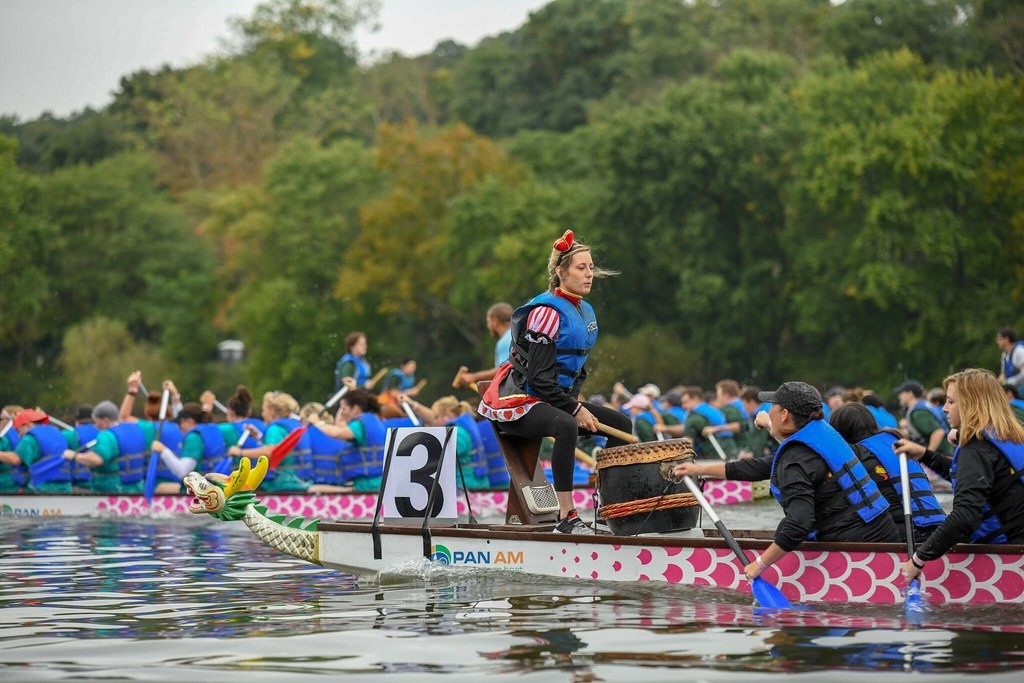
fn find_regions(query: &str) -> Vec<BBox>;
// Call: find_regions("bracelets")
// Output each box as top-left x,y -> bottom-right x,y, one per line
315,420 -> 324,428
172,394 -> 180,400
912,552 -> 926,567
72,452 -> 78,462
756,556 -> 767,570
128,387 -> 138,396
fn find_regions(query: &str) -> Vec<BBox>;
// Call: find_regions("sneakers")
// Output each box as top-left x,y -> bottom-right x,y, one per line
590,519 -> 611,532
552,509 -> 595,535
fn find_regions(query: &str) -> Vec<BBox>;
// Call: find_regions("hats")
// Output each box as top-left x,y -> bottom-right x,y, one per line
622,394 -> 650,411
172,410 -> 196,422
14,408 -> 48,428
758,382 -> 822,417
639,383 -> 661,399
894,383 -> 923,397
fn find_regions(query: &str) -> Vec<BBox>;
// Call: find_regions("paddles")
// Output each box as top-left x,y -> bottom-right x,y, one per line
0,412 -> 17,439
136,369 -> 149,397
621,386 -> 633,399
36,405 -> 74,431
269,380 -> 350,469
450,364 -> 596,469
705,432 -> 728,460
213,399 -> 228,414
399,395 -> 422,427
668,471 -> 793,609
893,441 -> 923,612
143,382 -> 170,504
29,439 -> 98,487
289,413 -> 301,422
653,422 -> 665,441
213,429 -> 252,475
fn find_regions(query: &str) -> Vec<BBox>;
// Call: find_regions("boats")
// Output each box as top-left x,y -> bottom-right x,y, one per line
184,424 -> 1023,639
0,475 -> 772,517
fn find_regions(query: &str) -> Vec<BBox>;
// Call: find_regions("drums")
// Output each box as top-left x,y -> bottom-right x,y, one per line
596,437 -> 701,536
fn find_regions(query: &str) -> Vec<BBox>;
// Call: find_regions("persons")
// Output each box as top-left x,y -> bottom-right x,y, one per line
475,230 -> 632,538
0,328 -> 511,496
462,302 -> 515,385
995,328 -> 1024,400
573,367 -> 1024,585
